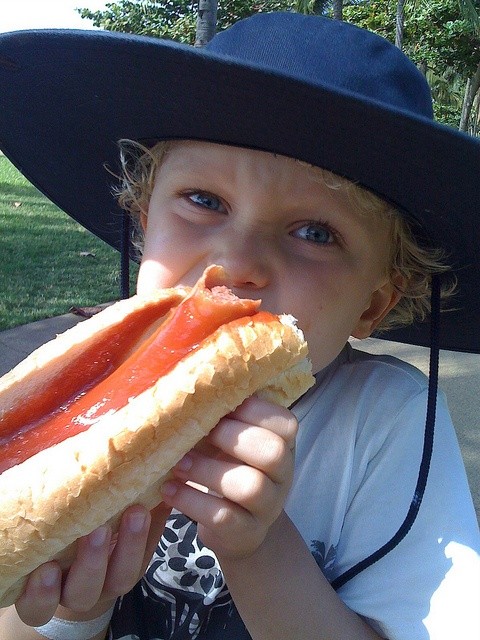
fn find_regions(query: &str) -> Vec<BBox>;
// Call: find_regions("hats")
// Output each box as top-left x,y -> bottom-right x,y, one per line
1,10 -> 480,355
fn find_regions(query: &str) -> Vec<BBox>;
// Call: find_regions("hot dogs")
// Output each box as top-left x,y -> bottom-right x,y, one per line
0,264 -> 318,610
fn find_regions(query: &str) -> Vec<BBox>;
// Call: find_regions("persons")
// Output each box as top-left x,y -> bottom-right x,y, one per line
0,12 -> 480,638
